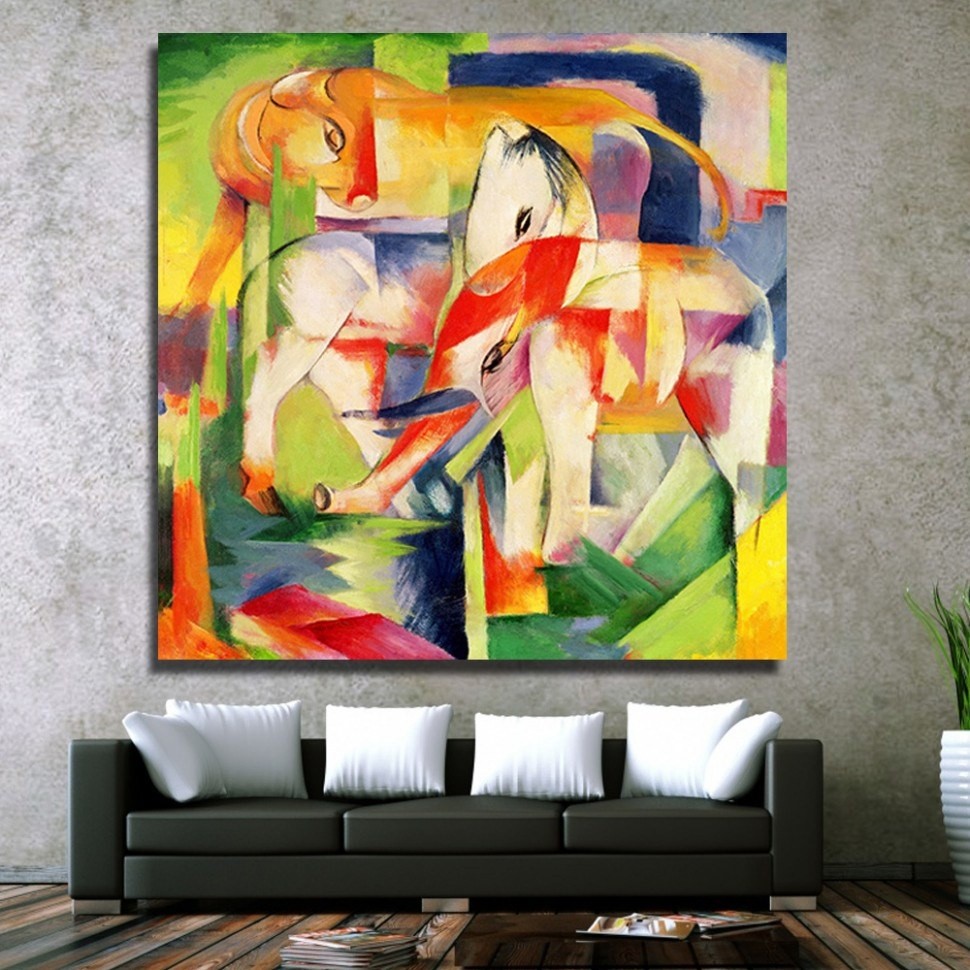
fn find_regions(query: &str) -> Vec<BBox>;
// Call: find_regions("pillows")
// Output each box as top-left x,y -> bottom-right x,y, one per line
164,698 -> 308,801
322,705 -> 453,801
468,712 -> 608,801
704,710 -> 783,800
618,693 -> 749,799
121,711 -> 227,805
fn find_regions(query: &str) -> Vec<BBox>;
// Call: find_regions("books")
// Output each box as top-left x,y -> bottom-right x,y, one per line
280,928 -> 428,970
576,910 -> 783,939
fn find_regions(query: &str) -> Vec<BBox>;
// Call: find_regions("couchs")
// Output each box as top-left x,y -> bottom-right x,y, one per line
67,731 -> 824,915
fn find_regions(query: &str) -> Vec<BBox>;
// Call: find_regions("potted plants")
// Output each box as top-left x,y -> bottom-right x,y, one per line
898,573 -> 969,908
